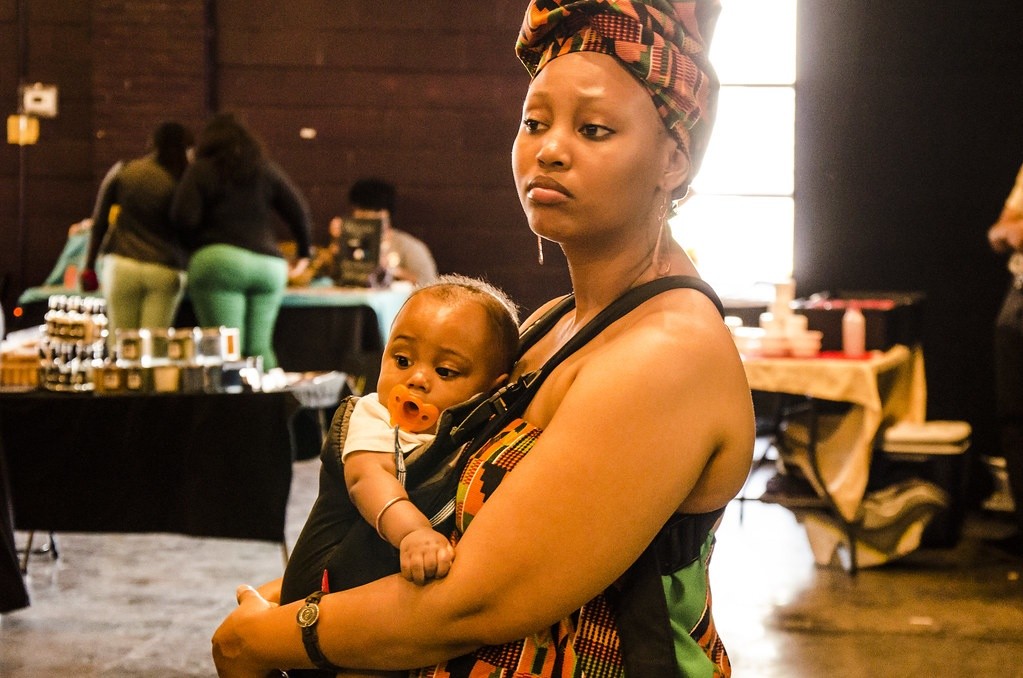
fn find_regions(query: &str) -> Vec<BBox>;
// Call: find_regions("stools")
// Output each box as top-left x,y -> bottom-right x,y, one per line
874,418 -> 976,550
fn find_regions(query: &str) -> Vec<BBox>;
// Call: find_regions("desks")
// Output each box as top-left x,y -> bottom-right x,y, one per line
737,345 -> 929,576
0,375 -> 354,570
20,283 -> 413,423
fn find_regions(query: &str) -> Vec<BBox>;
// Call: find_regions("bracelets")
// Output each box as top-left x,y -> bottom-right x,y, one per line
375,497 -> 410,542
296,591 -> 339,672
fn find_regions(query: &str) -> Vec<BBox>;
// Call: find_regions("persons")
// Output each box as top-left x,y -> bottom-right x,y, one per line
988,164 -> 1023,552
83,0 -> 754,678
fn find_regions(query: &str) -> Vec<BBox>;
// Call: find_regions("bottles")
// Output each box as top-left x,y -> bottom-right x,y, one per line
842,301 -> 866,357
36,294 -> 108,394
